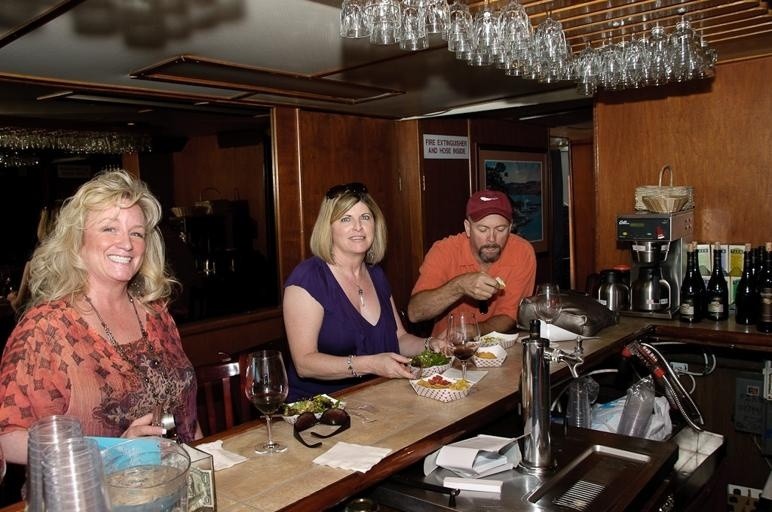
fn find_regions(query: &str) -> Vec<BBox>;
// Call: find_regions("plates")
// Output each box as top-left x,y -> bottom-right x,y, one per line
471,331 -> 520,368
404,350 -> 475,401
282,393 -> 346,424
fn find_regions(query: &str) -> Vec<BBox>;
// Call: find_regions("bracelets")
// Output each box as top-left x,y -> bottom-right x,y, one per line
423,336 -> 434,351
346,354 -> 363,378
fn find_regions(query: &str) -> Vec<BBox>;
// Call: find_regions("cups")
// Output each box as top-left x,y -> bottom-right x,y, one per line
27,414 -> 108,512
563,308 -> 587,322
569,378 -> 654,438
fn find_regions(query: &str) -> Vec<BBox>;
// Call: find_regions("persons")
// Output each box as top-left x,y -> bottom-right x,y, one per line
7,200 -> 56,319
408,188 -> 536,346
0,167 -> 204,502
283,184 -> 454,414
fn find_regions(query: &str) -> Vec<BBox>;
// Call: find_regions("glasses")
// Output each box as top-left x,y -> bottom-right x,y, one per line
292,407 -> 351,448
325,182 -> 368,198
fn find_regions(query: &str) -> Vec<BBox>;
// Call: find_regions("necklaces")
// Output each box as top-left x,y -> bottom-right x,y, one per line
350,275 -> 364,294
83,289 -> 181,444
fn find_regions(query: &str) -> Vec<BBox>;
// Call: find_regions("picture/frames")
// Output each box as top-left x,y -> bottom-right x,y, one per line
474,142 -> 554,258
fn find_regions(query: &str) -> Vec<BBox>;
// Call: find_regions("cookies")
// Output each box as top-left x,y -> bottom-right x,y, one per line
495,277 -> 506,290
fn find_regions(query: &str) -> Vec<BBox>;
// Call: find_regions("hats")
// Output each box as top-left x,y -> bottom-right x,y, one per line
466,189 -> 513,224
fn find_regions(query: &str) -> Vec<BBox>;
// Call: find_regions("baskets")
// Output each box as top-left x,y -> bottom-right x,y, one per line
634,164 -> 696,214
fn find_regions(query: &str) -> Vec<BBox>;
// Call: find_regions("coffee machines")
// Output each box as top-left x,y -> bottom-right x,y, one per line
616,210 -> 699,318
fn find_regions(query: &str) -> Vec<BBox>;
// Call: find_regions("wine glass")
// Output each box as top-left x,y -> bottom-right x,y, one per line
518,282 -> 562,357
339,0 -> 717,97
447,313 -> 481,384
243,352 -> 288,455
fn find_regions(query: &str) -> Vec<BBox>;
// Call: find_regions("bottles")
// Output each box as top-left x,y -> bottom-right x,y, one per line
203,258 -> 217,275
345,499 -> 380,512
679,241 -> 772,333
585,264 -> 630,313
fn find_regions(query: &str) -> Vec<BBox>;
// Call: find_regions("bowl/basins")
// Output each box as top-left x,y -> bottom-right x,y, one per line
95,435 -> 192,511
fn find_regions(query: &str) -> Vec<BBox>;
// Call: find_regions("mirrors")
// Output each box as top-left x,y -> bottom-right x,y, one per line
1,76 -> 284,361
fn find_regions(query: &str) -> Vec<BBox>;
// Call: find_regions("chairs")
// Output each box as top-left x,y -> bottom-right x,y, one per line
199,355 -> 254,436
